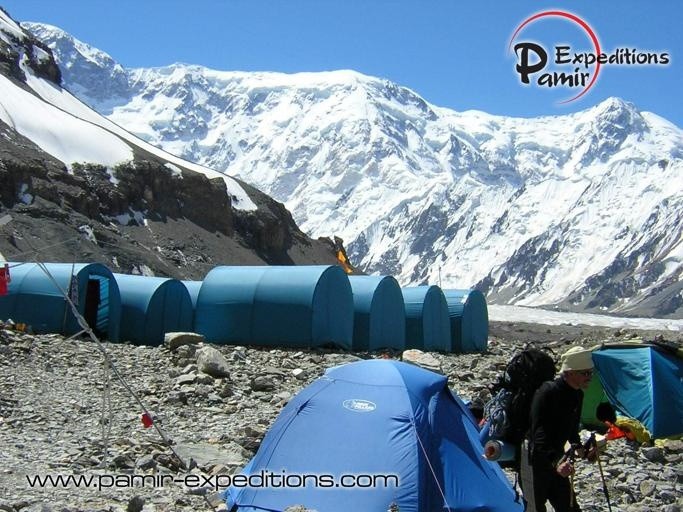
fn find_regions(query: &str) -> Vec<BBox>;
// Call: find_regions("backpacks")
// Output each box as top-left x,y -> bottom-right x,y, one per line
614,416 -> 651,443
504,342 -> 559,445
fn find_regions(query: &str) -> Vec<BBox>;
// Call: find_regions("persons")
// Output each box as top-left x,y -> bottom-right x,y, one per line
512,345 -> 599,512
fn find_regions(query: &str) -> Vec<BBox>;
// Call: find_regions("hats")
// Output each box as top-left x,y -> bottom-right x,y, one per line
562,346 -> 593,371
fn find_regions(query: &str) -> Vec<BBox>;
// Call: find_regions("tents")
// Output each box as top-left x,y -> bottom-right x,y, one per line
574,340 -> 683,442
214,356 -> 527,512
0,259 -> 491,353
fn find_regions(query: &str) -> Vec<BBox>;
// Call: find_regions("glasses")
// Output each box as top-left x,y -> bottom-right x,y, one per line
571,371 -> 593,377
